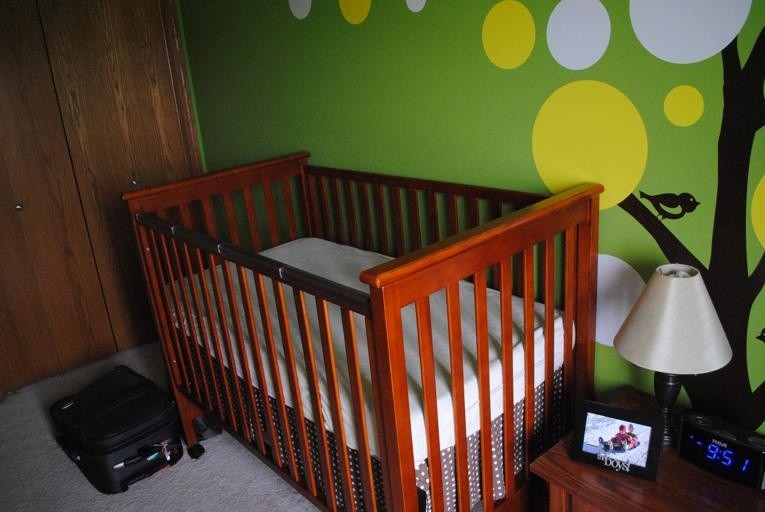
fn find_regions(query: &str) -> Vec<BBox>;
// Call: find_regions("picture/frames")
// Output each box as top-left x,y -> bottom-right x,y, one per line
575,399 -> 668,482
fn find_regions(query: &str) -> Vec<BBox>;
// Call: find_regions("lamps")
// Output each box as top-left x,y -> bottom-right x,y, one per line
612,263 -> 735,446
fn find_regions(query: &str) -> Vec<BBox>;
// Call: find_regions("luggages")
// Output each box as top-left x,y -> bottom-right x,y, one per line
48,363 -> 184,495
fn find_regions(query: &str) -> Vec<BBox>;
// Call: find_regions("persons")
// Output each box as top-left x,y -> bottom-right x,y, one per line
611,424 -> 632,444
627,424 -> 637,438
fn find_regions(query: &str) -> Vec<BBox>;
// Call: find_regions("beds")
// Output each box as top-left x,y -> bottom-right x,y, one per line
121,148 -> 606,508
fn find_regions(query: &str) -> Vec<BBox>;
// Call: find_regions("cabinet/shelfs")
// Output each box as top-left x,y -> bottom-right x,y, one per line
1,2 -> 210,402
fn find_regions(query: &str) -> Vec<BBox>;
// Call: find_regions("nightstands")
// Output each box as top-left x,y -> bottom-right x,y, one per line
529,401 -> 765,510
